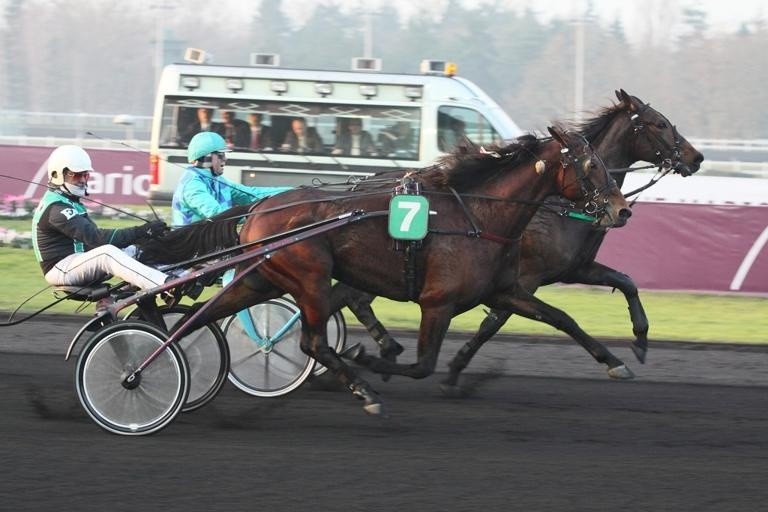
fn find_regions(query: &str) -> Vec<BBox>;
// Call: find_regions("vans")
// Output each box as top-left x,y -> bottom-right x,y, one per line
146,47 -> 528,209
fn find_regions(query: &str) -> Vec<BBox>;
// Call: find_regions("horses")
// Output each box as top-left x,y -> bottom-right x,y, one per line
140,124 -> 637,418
329,87 -> 705,401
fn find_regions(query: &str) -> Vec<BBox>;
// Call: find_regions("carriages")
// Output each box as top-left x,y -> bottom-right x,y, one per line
144,86 -> 705,400
49,125 -> 635,438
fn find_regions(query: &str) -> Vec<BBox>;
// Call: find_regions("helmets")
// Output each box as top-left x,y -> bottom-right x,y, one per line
188,132 -> 228,163
47,146 -> 94,185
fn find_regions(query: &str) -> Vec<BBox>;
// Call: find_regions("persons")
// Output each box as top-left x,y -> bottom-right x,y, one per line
28,146 -> 226,313
438,118 -> 475,157
278,117 -> 326,158
328,117 -> 380,159
174,106 -> 221,153
213,110 -> 252,156
245,113 -> 281,151
167,133 -> 303,231
391,119 -> 418,160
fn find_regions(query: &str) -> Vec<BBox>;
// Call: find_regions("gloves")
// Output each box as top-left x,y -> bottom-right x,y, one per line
136,221 -> 166,240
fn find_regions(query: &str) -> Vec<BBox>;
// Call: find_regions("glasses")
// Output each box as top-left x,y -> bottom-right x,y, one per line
67,174 -> 89,181
213,150 -> 224,159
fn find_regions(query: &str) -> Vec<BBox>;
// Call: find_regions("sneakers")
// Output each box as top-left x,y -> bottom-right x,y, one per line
162,258 -> 220,309
